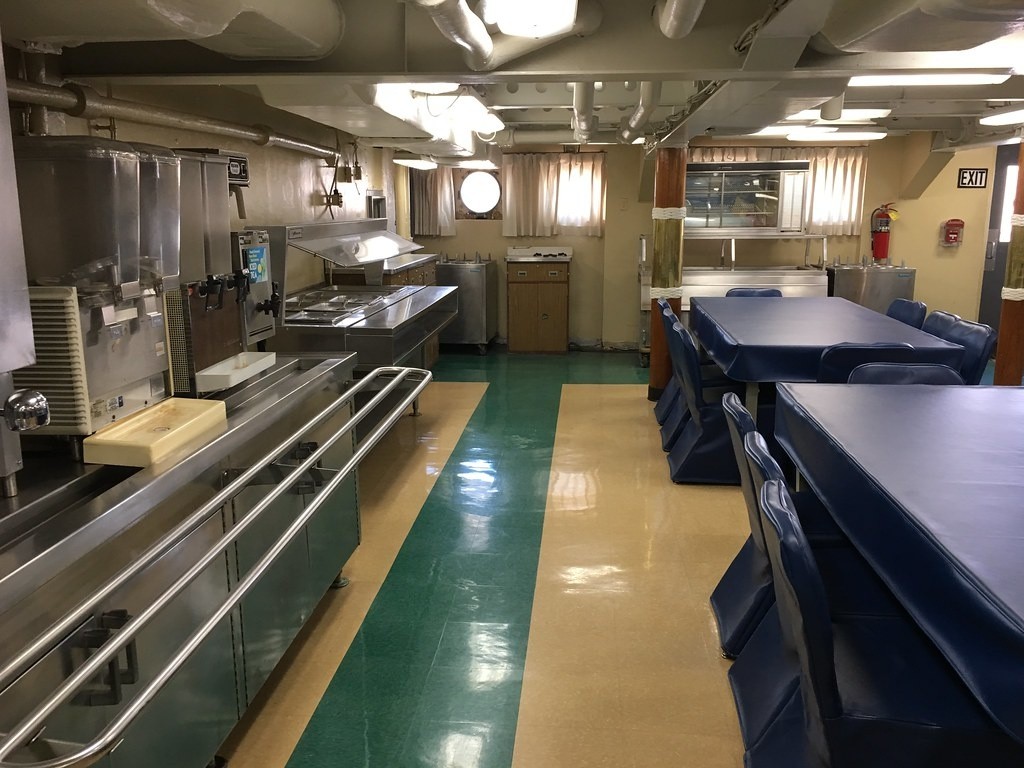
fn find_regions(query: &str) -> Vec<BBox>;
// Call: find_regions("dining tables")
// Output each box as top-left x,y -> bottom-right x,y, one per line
689,294 -> 967,493
773,383 -> 1024,717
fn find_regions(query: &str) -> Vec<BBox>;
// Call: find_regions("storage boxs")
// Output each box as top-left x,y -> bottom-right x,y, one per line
201,151 -> 234,277
168,149 -> 207,288
15,135 -> 144,303
121,141 -> 184,286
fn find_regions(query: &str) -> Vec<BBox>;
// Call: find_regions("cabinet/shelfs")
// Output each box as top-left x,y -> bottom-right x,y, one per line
505,253 -> 571,353
635,234 -> 829,361
244,215 -> 459,417
223,373 -> 364,706
434,259 -> 498,356
0,458 -> 241,768
684,159 -> 810,234
833,255 -> 917,315
328,253 -> 441,378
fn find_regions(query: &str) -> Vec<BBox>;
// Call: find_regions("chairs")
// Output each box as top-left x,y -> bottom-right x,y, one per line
724,430 -> 914,752
847,360 -> 966,387
742,478 -> 1023,768
665,321 -> 779,486
659,308 -> 734,439
651,296 -> 729,426
708,392 -> 855,661
724,287 -> 784,298
816,340 -> 919,384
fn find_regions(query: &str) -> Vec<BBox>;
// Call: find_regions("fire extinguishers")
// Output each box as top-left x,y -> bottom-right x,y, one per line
870,201 -> 898,259
